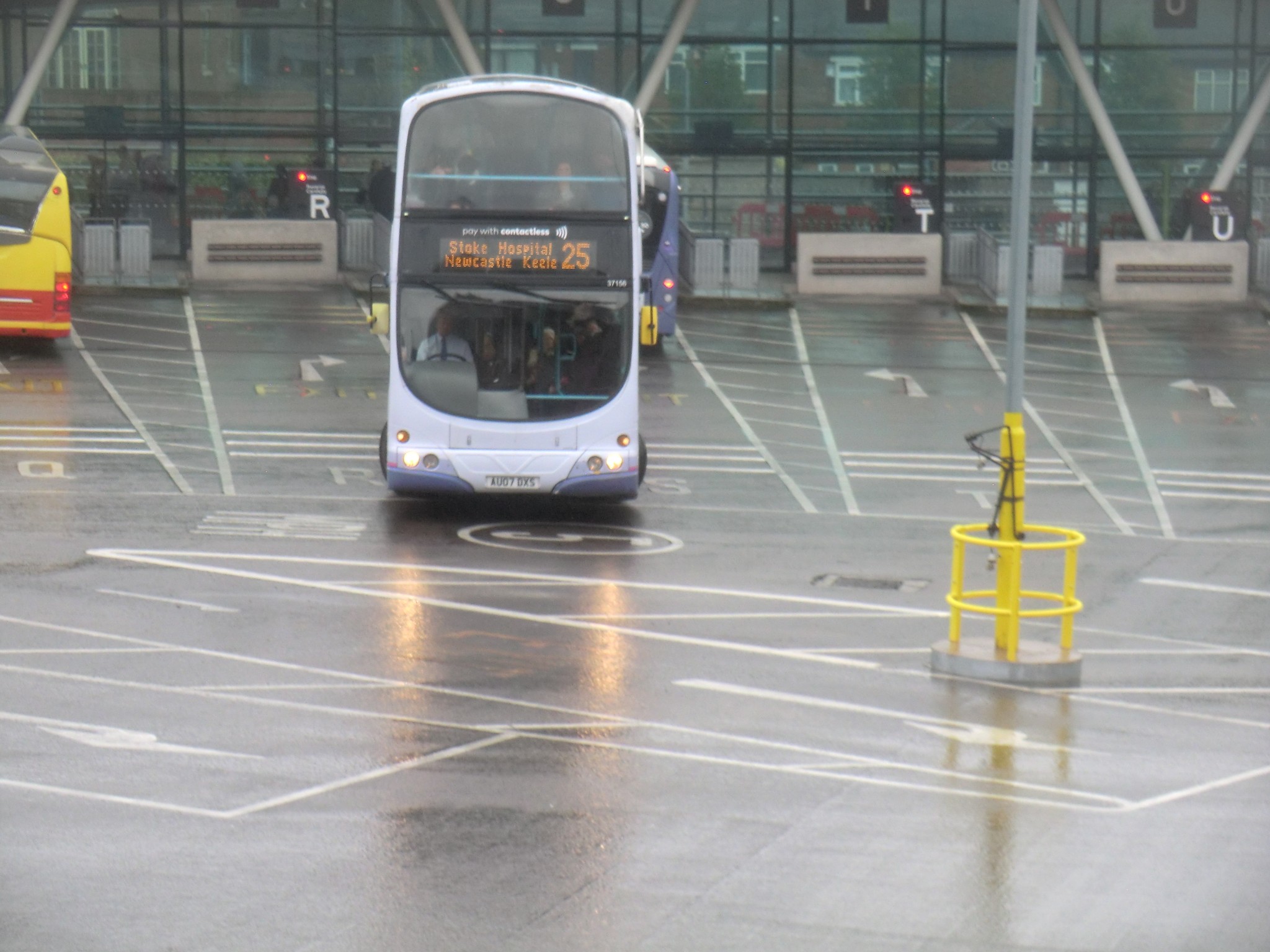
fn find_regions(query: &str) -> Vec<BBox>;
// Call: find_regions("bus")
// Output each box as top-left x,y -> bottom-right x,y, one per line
368,72 -> 659,504
0,123 -> 74,351
633,132 -> 681,351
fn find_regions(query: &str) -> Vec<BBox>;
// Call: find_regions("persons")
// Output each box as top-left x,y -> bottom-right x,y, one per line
88,145 -> 396,223
416,314 -> 474,365
480,304 -> 624,407
419,160 -> 594,213
